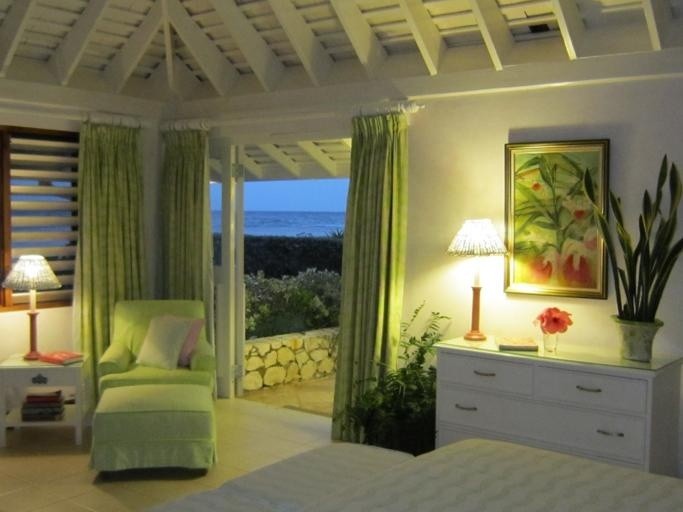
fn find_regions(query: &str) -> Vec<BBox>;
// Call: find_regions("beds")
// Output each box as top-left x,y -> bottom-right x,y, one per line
286,439 -> 683,512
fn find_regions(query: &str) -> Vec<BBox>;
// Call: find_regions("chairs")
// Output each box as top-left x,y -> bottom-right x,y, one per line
98,299 -> 215,396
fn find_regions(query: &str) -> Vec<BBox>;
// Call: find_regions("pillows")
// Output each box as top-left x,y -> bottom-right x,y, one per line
136,314 -> 193,369
178,319 -> 205,366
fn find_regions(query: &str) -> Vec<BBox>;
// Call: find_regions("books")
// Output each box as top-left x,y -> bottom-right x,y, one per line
493,333 -> 539,352
41,351 -> 84,365
19,392 -> 65,420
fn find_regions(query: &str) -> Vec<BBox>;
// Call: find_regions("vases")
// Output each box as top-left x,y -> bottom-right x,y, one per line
543,330 -> 557,352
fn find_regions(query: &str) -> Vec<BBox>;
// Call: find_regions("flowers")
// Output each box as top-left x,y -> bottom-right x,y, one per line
536,306 -> 573,335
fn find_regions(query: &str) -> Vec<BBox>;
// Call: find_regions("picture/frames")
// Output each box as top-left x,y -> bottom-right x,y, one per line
505,139 -> 607,300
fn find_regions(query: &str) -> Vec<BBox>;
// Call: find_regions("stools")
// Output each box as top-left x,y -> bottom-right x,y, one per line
87,383 -> 218,478
148,438 -> 414,512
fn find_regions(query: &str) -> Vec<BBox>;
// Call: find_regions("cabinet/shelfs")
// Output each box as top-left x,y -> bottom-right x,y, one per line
431,338 -> 683,474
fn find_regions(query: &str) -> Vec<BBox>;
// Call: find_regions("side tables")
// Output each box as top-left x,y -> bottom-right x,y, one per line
0,352 -> 92,447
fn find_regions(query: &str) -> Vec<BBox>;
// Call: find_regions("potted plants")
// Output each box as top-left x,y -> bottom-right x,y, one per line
581,153 -> 683,362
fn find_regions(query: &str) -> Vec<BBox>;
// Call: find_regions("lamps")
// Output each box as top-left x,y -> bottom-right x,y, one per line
446,217 -> 508,341
1,255 -> 63,360
